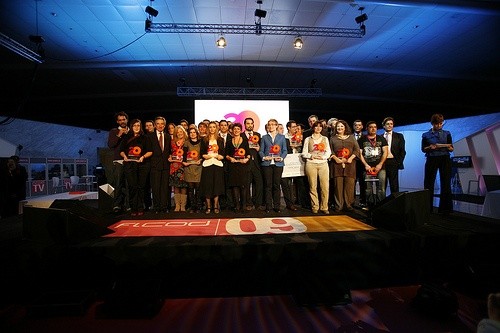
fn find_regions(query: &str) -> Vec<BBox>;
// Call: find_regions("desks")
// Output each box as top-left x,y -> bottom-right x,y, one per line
82,176 -> 95,192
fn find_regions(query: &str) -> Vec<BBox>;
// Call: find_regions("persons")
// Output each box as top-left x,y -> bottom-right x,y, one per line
421,113 -> 455,210
0,156 -> 28,218
108,114 -> 407,216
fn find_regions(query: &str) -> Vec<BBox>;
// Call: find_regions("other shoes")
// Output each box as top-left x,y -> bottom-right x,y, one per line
358,202 -> 367,208
220,205 -> 248,214
335,207 -> 356,213
286,204 -> 297,211
114,208 -> 145,218
298,204 -> 311,209
146,207 -> 152,212
202,204 -> 220,214
311,208 -> 330,214
154,208 -> 168,215
246,209 -> 282,218
436,211 -> 451,216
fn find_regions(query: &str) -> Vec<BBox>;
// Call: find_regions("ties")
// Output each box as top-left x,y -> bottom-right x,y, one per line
222,134 -> 225,138
159,132 -> 163,151
248,132 -> 252,139
385,133 -> 389,144
356,133 -> 360,144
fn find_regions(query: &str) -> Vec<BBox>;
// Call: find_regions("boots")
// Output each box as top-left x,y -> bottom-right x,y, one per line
173,193 -> 187,211
188,191 -> 202,214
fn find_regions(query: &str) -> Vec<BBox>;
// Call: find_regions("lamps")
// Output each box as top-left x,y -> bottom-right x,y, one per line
145,6 -> 159,17
255,8 -> 267,18
217,37 -> 226,49
355,13 -> 368,24
294,35 -> 303,49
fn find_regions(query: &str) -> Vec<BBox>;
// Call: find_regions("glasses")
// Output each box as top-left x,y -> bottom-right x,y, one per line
132,124 -> 140,127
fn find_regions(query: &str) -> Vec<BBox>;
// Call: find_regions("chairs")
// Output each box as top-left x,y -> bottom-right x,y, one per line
52,177 -> 65,193
70,176 -> 83,192
